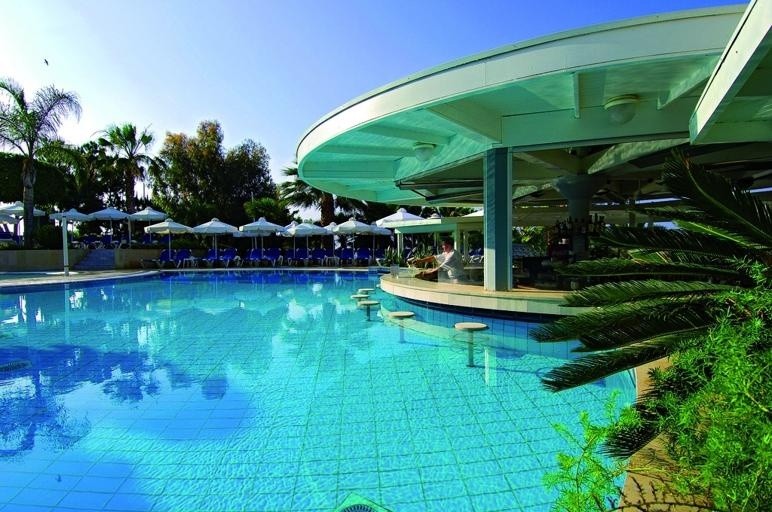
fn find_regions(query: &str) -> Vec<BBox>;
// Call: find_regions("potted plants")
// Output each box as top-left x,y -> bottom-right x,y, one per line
382,246 -> 403,276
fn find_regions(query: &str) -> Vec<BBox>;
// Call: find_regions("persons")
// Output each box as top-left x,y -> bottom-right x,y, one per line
414,236 -> 467,285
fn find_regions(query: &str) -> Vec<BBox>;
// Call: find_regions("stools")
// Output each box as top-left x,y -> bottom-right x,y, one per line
388,311 -> 415,344
351,283 -> 381,321
453,321 -> 489,367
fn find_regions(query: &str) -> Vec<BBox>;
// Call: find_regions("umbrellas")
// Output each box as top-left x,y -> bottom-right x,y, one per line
0,200 -> 47,249
88,204 -> 129,247
192,216 -> 239,261
144,217 -> 191,260
125,205 -> 169,246
237,205 -> 428,267
0,214 -> 18,225
50,207 -> 93,248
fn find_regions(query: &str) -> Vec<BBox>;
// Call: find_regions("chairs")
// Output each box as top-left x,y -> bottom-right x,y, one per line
141,247 -> 432,271
463,254 -> 484,265
70,231 -> 175,249
1,226 -> 23,247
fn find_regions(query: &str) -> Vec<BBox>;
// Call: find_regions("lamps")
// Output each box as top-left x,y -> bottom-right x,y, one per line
604,93 -> 638,124
412,142 -> 435,161
